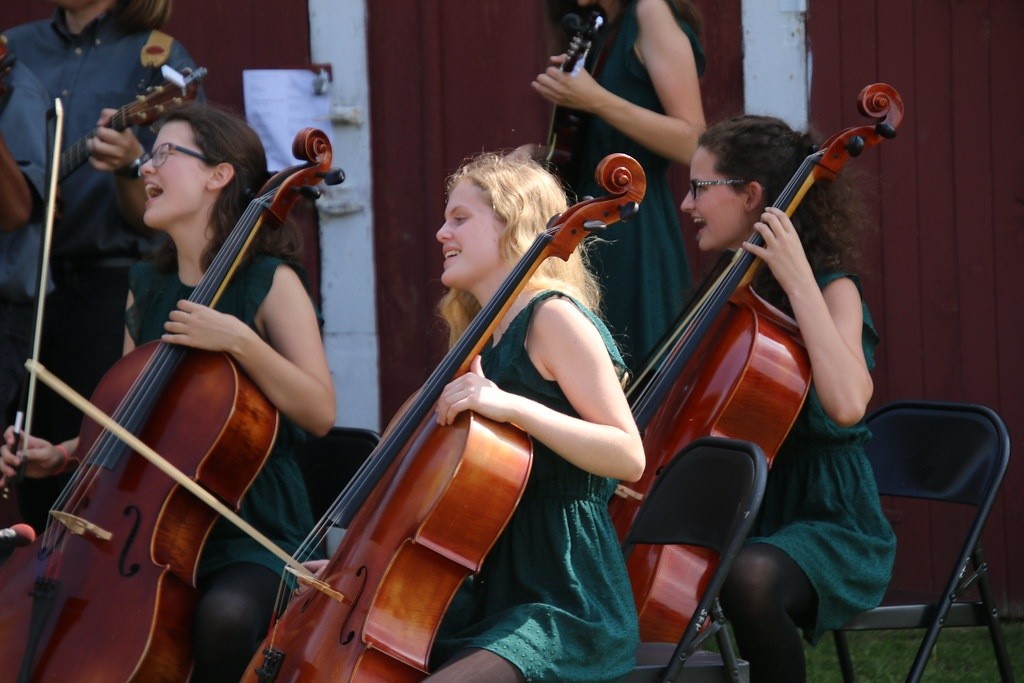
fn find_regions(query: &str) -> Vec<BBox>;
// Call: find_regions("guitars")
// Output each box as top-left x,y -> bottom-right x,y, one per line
43,64 -> 209,222
543,8 -> 608,178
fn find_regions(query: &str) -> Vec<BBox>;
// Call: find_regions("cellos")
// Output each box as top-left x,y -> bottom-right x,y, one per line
1,125 -> 347,682
239,151 -> 649,683
596,78 -> 909,645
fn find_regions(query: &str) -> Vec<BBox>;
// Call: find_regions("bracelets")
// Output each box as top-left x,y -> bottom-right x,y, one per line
51,445 -> 68,476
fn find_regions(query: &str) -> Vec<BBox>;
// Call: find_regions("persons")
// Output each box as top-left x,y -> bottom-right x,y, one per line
0,58 -> 54,438
681,115 -> 897,683
1,106 -> 336,682
505,0 -> 708,376
297,152 -> 646,683
0,0 -> 206,536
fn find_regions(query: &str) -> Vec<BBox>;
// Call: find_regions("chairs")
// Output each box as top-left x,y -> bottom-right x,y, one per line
302,426 -> 381,524
608,435 -> 768,683
810,398 -> 1014,683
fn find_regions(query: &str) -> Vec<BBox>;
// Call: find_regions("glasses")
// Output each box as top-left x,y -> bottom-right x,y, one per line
688,179 -> 748,200
137,143 -> 216,176
